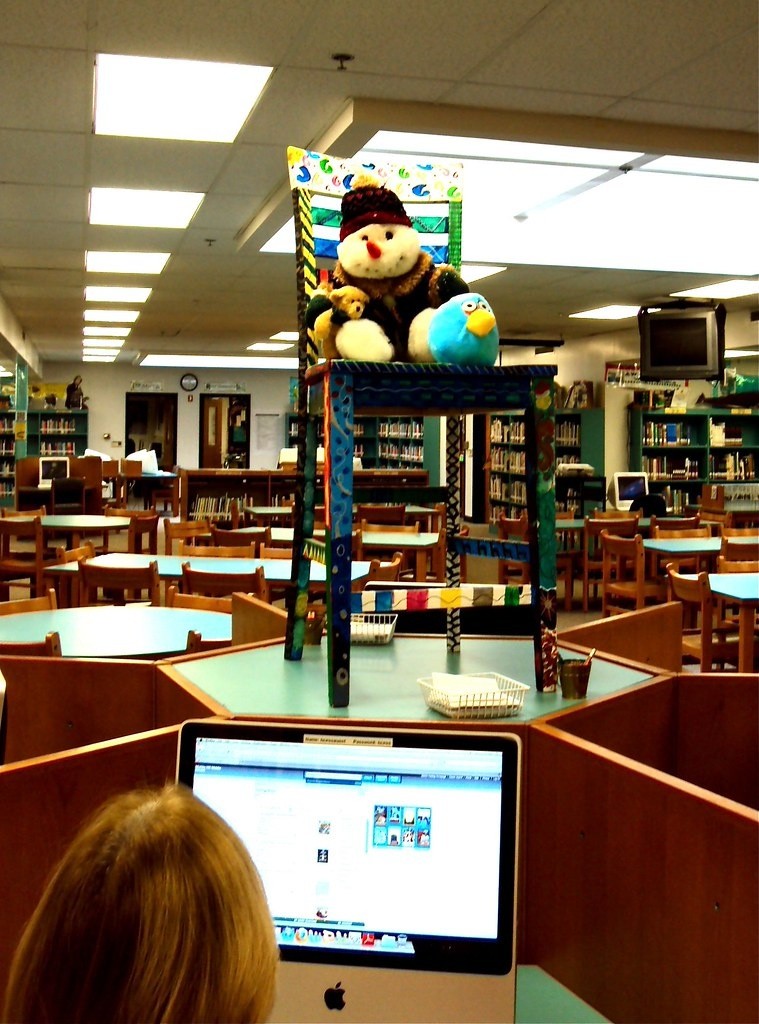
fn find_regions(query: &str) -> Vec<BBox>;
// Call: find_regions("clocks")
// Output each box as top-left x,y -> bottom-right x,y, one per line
181,374 -> 198,391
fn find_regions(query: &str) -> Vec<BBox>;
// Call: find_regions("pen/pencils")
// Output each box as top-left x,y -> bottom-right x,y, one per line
557,652 -> 564,664
585,647 -> 596,664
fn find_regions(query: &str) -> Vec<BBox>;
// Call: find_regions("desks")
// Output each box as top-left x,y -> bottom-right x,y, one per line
43,552 -> 394,603
2,514 -> 131,550
555,518 -> 724,597
243,500 -> 441,527
1,606 -> 231,658
684,502 -> 759,530
236,525 -> 438,582
665,571 -> 759,677
12,454 -> 102,513
642,537 -> 759,584
141,471 -> 177,510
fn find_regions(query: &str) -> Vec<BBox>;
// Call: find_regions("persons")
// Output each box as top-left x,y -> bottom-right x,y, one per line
0,785 -> 279,1024
65,375 -> 82,409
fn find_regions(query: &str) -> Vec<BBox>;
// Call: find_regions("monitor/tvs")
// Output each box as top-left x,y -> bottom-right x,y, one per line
39,457 -> 69,484
174,718 -> 522,1024
608,472 -> 649,511
640,309 -> 718,380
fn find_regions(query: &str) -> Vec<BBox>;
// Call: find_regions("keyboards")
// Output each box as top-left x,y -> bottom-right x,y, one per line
38,484 -> 51,488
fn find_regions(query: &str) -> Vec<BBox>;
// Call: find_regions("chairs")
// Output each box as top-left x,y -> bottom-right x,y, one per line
1,453 -> 759,677
285,147 -> 559,710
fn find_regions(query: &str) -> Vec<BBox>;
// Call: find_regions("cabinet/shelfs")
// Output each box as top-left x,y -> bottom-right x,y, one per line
486,408 -> 604,555
633,408 -> 759,515
0,358 -> 89,513
180,466 -> 429,526
286,410 -> 439,502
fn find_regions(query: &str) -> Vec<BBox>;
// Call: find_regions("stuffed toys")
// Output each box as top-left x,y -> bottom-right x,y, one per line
306,170 -> 470,363
430,293 -> 500,365
313,286 -> 369,359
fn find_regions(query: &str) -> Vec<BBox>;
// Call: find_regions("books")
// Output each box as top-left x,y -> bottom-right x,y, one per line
555,421 -> 580,466
353,424 -> 364,458
40,418 -> 75,456
643,418 -> 755,514
0,418 -> 16,498
271,493 -> 294,520
380,423 -> 423,462
194,494 -> 253,520
490,417 -> 527,519
